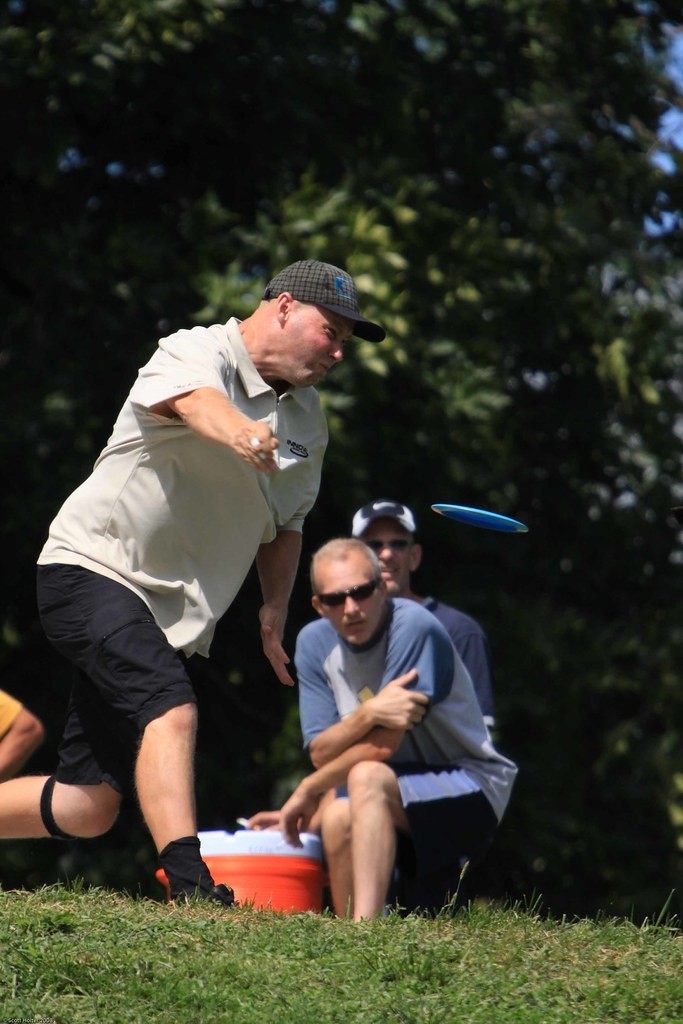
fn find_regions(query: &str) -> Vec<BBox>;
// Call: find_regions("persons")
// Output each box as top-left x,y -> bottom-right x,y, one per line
278,537 -> 518,924
0,689 -> 45,784
246,499 -> 495,830
0,260 -> 386,910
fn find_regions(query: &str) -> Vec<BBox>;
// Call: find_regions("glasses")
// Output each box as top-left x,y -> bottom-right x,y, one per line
319,577 -> 380,605
366,540 -> 408,550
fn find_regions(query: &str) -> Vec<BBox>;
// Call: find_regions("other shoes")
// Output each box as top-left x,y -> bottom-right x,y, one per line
170,884 -> 237,907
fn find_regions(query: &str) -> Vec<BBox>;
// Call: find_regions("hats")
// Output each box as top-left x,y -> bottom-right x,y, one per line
352,499 -> 416,537
261,259 -> 386,342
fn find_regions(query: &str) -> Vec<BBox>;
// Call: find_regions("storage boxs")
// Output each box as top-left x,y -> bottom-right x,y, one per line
156,830 -> 327,914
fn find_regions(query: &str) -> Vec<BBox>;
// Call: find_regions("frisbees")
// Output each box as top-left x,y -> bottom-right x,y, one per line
431,504 -> 529,534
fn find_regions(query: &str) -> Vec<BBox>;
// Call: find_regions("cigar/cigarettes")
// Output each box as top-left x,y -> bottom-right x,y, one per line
237,817 -> 261,830
